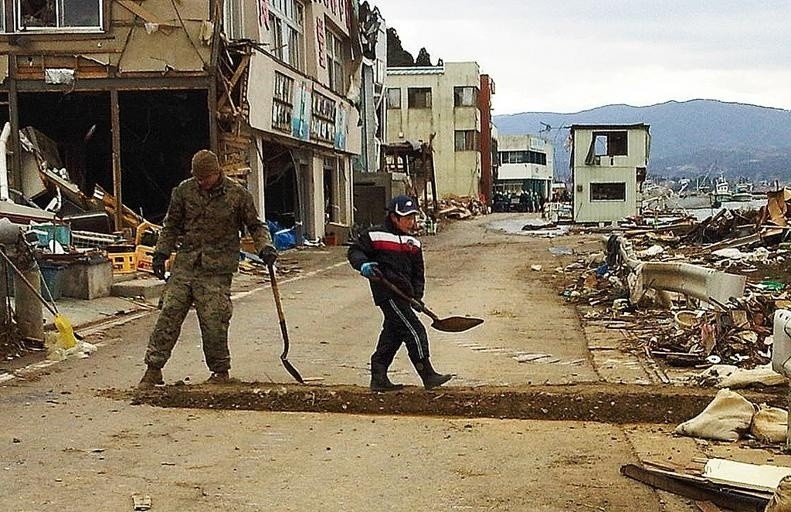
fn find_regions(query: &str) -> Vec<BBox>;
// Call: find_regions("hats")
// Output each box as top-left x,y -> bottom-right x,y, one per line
389,194 -> 422,217
191,148 -> 219,178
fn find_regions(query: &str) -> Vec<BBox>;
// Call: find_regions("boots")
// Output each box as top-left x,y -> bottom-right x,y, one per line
416,362 -> 452,389
369,369 -> 402,391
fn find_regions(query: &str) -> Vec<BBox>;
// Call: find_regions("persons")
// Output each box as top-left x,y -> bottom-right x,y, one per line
346,194 -> 451,392
135,148 -> 280,383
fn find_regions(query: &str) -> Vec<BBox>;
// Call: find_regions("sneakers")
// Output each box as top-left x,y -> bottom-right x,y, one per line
138,364 -> 164,389
205,371 -> 230,384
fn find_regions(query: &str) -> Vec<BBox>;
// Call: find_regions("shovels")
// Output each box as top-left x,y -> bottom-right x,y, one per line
360,268 -> 484,332
1,250 -> 76,338
268,261 -> 304,384
20,230 -> 84,341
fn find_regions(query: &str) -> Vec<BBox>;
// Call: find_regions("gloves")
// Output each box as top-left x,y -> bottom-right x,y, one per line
260,246 -> 279,264
412,298 -> 426,312
359,262 -> 380,281
152,252 -> 170,280
